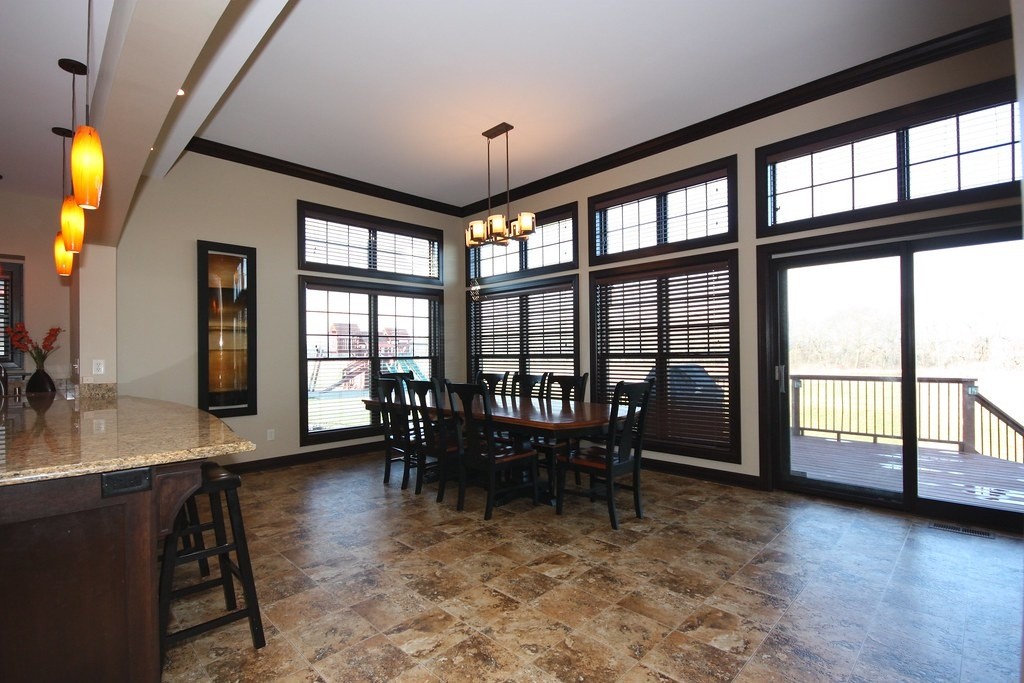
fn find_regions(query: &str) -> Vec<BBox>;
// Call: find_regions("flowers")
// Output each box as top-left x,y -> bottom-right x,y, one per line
7,415 -> 59,464
5,322 -> 66,369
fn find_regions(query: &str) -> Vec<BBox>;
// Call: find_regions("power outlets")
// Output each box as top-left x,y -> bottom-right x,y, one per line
93,359 -> 104,375
93,418 -> 105,433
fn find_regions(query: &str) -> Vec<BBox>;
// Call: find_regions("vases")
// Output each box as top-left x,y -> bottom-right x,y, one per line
26,367 -> 56,392
26,392 -> 57,415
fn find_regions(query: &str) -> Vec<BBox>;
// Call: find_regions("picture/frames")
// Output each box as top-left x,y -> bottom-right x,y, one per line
197,239 -> 258,418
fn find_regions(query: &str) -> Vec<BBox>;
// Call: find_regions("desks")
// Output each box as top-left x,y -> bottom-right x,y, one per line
362,390 -> 655,507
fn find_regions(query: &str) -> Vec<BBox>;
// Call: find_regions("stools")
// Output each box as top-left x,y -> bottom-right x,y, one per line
157,461 -> 265,673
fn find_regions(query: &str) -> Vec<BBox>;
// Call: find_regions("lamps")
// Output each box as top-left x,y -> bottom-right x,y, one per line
72,0 -> 104,209
465,121 -> 536,249
51,126 -> 75,277
59,59 -> 88,253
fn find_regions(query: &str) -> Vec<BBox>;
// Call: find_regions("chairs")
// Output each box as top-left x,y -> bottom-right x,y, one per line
375,369 -> 656,530
